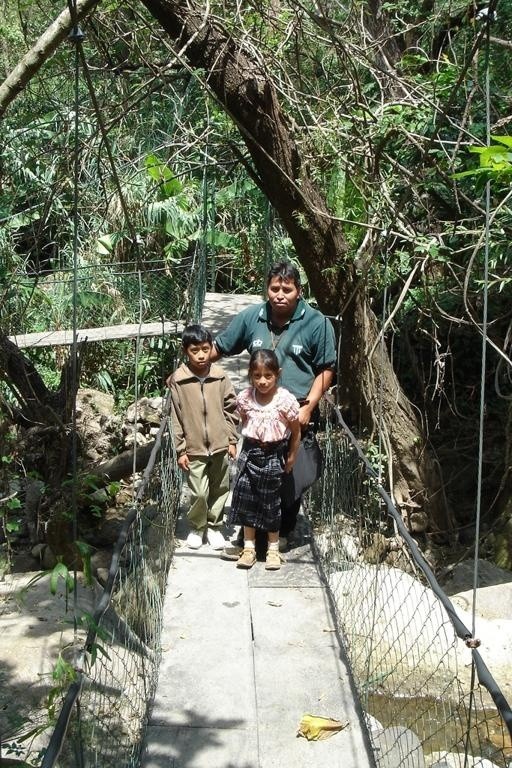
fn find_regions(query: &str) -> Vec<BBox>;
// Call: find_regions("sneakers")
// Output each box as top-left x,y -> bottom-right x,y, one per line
185,527 -> 205,550
221,544 -> 245,560
279,536 -> 288,551
265,549 -> 282,570
236,548 -> 258,568
206,527 -> 226,550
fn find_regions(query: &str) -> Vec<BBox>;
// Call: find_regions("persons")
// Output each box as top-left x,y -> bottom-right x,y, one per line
234,348 -> 302,572
166,323 -> 241,554
165,259 -> 340,556
